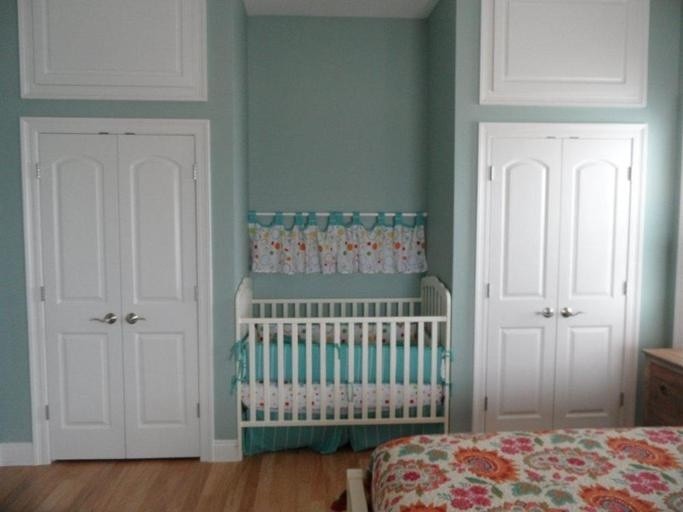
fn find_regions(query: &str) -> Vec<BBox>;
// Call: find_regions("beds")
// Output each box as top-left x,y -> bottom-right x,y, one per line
347,424 -> 683,512
233,274 -> 452,461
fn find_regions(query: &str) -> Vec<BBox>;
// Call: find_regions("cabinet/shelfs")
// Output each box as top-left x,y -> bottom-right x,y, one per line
641,347 -> 683,427
